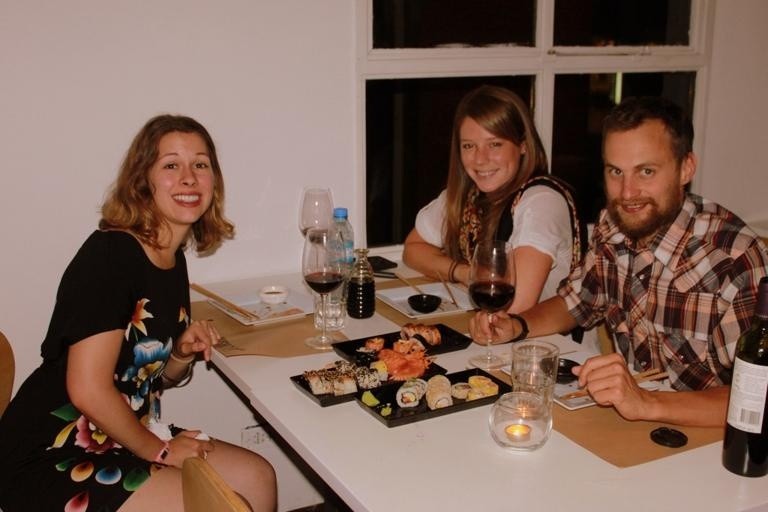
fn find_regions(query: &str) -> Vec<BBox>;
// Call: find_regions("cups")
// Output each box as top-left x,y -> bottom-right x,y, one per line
508,337 -> 562,411
486,391 -> 554,451
312,270 -> 342,328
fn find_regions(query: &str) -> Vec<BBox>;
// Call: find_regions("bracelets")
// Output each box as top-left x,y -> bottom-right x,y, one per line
448,261 -> 459,283
509,314 -> 528,342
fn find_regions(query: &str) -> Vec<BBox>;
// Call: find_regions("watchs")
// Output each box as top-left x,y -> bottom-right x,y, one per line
155,441 -> 170,463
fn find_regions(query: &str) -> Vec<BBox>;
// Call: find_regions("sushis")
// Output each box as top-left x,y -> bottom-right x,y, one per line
304,323 -> 498,409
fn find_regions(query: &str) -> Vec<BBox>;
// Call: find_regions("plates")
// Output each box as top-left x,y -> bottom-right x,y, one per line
373,281 -> 476,323
289,321 -> 513,430
212,284 -> 315,330
362,244 -> 424,285
498,349 -> 664,412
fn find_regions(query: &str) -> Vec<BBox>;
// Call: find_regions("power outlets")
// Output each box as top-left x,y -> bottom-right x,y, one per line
240,425 -> 273,449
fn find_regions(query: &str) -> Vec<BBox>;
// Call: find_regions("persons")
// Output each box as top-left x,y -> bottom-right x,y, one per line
465,93 -> 767,431
402,83 -> 588,315
1,114 -> 278,512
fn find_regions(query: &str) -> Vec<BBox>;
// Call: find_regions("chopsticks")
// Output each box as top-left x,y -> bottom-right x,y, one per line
559,367 -> 670,403
389,267 -> 458,311
191,281 -> 259,323
371,269 -> 397,280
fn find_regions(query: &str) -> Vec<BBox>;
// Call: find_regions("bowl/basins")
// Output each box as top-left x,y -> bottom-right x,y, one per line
539,357 -> 582,385
406,293 -> 443,314
258,285 -> 294,304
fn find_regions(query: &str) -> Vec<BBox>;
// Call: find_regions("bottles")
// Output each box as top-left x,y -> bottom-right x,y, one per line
720,277 -> 766,479
328,206 -> 376,321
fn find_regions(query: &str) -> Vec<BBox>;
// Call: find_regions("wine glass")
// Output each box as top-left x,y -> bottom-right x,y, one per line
295,187 -> 345,351
468,240 -> 517,371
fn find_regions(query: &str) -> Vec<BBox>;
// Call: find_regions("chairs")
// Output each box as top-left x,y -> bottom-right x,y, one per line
179,454 -> 264,512
0,327 -> 18,425
